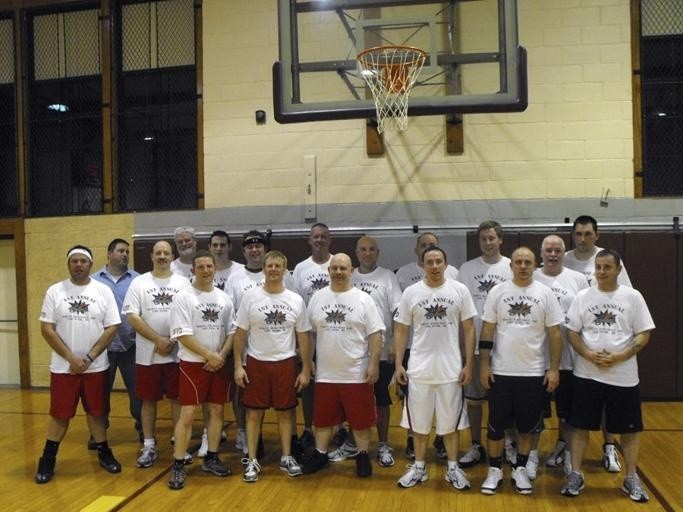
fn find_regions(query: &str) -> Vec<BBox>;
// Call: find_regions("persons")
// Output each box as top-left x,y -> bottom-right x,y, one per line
89,238 -> 158,448
560,250 -> 656,502
121,215 -> 633,496
34,244 -> 121,483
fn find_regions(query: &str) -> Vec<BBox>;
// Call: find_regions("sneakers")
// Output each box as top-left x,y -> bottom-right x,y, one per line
563,449 -> 572,475
197,434 -> 209,457
235,432 -> 265,482
36,456 -> 56,483
97,448 -> 121,473
405,446 -> 415,459
458,445 -> 486,463
355,449 -> 372,477
183,450 -> 192,463
432,436 -> 447,460
480,437 -> 539,494
169,464 -> 188,489
444,464 -> 470,489
136,447 -> 158,468
397,465 -> 428,488
377,439 -> 395,467
546,439 -> 566,467
602,443 -> 622,472
622,472 -> 649,502
560,470 -> 585,496
278,430 -> 330,476
332,430 -> 348,446
201,455 -> 232,476
327,440 -> 360,462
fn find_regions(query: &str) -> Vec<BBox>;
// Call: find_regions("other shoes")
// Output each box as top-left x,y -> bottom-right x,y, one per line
221,431 -> 227,441
88,436 -> 97,451
139,430 -> 156,445
